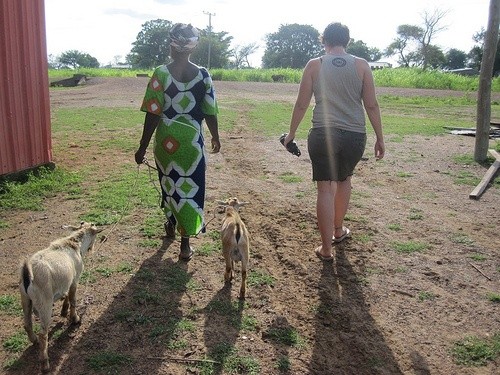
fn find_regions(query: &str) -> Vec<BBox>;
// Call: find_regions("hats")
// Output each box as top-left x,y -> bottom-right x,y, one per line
169,23 -> 200,53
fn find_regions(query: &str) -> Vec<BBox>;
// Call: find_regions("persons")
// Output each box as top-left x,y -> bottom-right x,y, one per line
283,22 -> 385,263
134,22 -> 222,260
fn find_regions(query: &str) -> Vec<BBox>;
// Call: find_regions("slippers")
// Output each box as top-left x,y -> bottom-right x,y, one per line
332,225 -> 350,242
315,245 -> 334,261
180,246 -> 194,262
163,220 -> 176,238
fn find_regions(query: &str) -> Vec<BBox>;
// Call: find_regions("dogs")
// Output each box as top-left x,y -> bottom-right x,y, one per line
16,218 -> 104,372
212,193 -> 255,302
